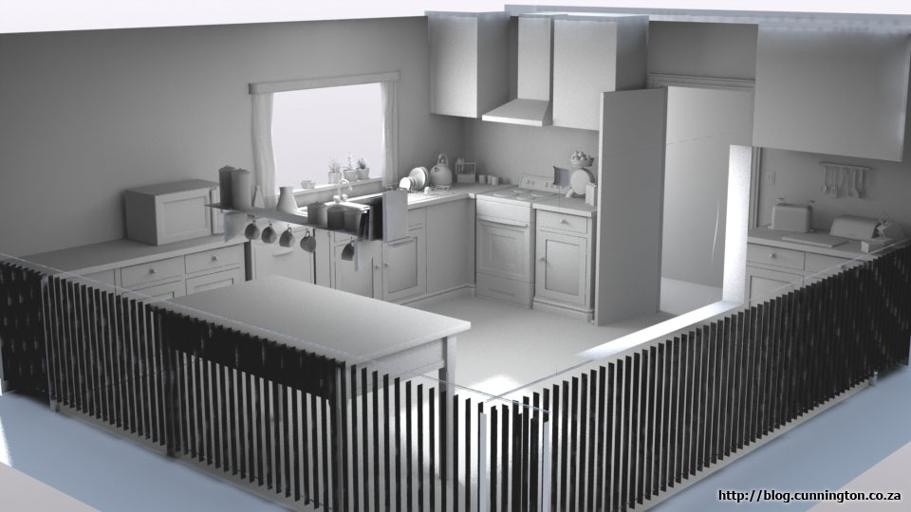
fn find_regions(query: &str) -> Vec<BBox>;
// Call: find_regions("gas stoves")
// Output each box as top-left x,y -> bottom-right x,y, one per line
476,187 -> 558,208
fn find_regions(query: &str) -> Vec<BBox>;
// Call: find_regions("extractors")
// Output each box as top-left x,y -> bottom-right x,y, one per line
481,17 -> 555,128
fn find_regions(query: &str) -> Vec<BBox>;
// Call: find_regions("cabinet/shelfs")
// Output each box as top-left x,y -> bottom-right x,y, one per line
748,246 -> 851,309
330,207 -> 429,306
80,245 -> 245,302
533,211 -> 594,321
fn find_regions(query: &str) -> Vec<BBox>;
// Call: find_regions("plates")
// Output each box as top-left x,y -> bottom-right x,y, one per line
400,167 -> 430,192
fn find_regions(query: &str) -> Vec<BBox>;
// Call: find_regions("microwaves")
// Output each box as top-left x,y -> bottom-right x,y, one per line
124,178 -> 224,246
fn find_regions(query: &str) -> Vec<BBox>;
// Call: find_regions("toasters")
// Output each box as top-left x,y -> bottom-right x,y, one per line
767,203 -> 813,233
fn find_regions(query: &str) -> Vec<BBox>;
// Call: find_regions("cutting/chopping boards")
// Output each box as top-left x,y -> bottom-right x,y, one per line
782,232 -> 848,248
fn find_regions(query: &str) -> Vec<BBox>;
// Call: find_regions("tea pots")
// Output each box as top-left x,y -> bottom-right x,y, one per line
430,153 -> 453,186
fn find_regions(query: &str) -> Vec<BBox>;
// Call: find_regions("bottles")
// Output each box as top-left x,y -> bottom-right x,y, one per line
218,165 -> 252,210
307,203 -> 345,231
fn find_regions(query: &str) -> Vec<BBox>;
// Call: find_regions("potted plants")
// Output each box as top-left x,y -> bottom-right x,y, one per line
328,151 -> 371,184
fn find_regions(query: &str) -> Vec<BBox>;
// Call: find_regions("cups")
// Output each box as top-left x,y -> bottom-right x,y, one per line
243,220 -> 356,261
480,175 -> 499,186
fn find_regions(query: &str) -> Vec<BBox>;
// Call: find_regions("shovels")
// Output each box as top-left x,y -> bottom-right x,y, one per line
848,169 -> 860,199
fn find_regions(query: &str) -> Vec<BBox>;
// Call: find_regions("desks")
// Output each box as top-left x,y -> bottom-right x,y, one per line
150,275 -> 472,511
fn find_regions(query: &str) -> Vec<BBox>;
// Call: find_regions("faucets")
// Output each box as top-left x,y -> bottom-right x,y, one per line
338,178 -> 354,201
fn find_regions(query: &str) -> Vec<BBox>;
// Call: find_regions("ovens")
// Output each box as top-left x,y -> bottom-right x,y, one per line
475,200 -> 536,308
